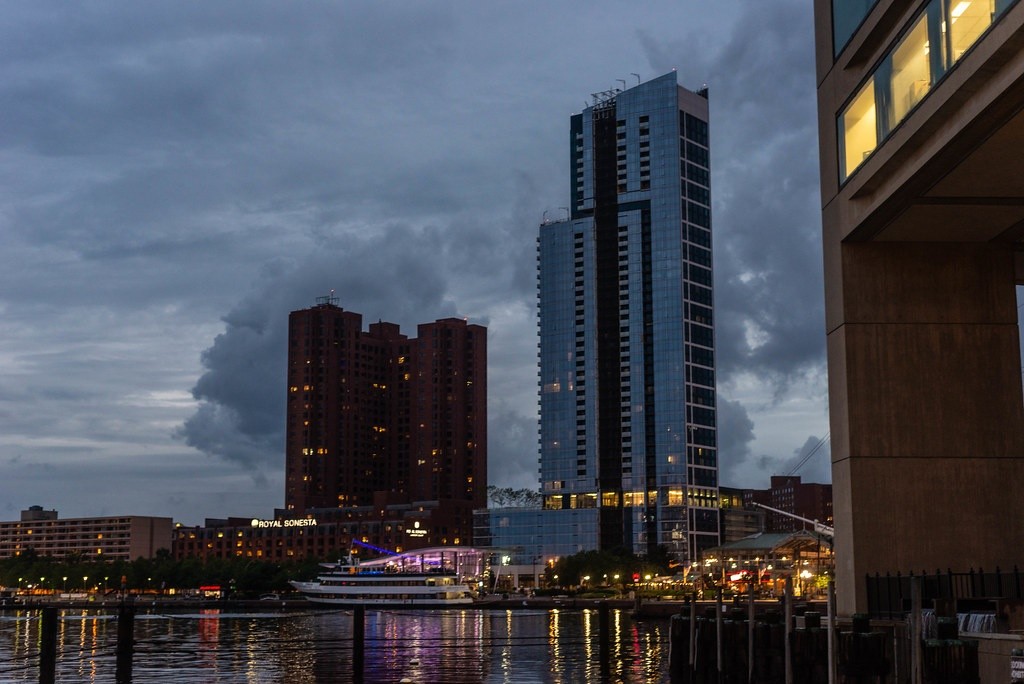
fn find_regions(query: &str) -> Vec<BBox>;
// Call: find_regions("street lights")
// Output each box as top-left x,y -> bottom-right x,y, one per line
40,577 -> 45,591
18,578 -> 22,590
104,576 -> 110,594
83,576 -> 88,593
63,576 -> 68,593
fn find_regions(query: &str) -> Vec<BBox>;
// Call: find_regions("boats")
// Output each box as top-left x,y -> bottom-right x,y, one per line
290,558 -> 475,609
